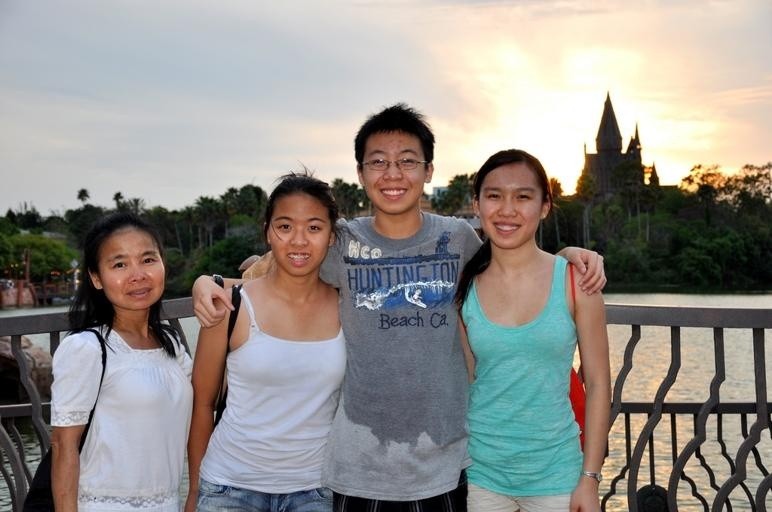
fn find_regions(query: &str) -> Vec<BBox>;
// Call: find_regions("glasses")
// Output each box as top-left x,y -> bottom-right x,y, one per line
362,158 -> 428,171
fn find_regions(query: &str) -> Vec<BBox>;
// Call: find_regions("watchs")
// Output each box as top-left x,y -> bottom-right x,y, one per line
582,471 -> 602,482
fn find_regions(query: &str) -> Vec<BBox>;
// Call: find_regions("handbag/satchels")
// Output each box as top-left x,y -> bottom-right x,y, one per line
556,361 -> 589,454
23,327 -> 106,512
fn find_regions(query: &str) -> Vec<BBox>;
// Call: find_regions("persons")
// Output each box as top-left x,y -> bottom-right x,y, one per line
192,102 -> 608,512
50,216 -> 226,512
185,175 -> 347,512
455,149 -> 610,512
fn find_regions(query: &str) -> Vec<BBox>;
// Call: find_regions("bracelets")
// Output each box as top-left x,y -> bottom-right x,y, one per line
213,274 -> 224,289
